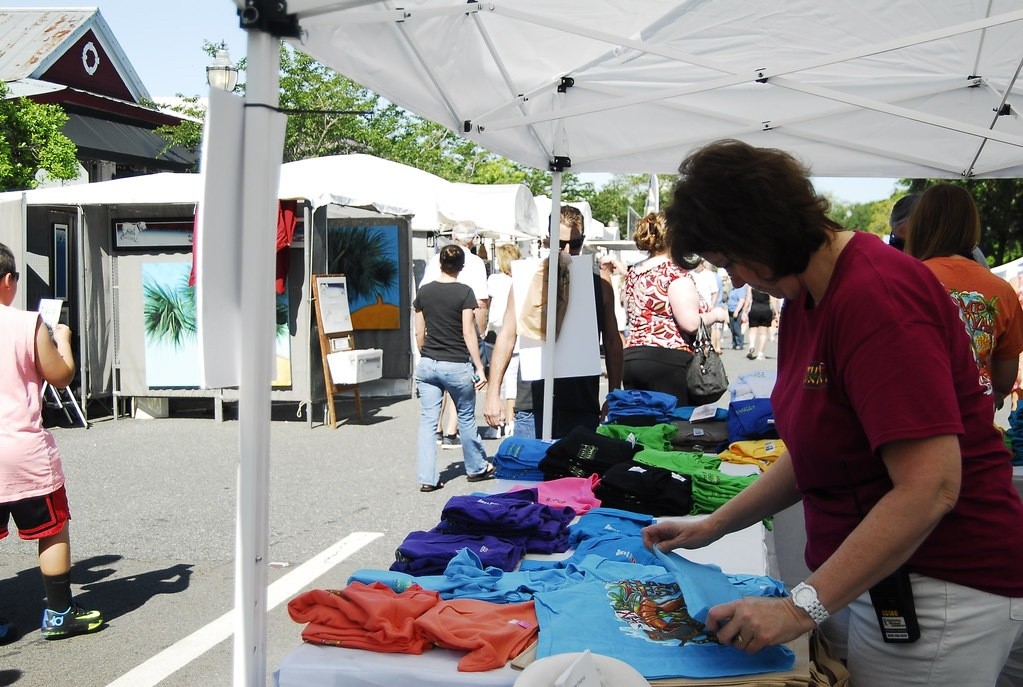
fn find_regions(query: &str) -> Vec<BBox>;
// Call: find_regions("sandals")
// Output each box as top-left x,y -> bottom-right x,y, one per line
467,463 -> 497,481
420,481 -> 443,491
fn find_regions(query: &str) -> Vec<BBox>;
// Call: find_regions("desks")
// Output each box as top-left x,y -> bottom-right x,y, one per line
272,453 -> 770,687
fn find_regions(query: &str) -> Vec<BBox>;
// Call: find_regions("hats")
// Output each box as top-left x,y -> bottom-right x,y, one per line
452,220 -> 479,238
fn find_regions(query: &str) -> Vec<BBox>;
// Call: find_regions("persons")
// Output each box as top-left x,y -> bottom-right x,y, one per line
419,220 -> 490,450
888,182 -> 1023,467
414,245 -> 499,494
0,243 -> 104,640
635,139 -> 1023,687
484,204 -> 624,439
623,212 -> 732,406
593,257 -> 783,361
487,243 -> 522,438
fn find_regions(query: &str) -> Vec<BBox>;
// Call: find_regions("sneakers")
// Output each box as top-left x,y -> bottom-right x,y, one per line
0,619 -> 19,645
41,601 -> 104,636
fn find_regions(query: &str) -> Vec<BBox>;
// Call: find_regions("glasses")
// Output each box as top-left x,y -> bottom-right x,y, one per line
0,271 -> 19,282
559,235 -> 585,249
889,231 -> 905,250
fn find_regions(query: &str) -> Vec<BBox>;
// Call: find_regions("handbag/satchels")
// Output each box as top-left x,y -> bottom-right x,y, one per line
508,625 -> 852,686
688,318 -> 729,405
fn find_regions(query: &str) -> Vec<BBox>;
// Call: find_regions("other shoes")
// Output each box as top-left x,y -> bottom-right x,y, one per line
436,432 -> 443,444
441,433 -> 462,448
736,345 -> 743,350
756,352 -> 767,360
747,347 -> 755,358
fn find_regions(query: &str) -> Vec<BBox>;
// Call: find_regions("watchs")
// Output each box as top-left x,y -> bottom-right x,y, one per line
788,580 -> 831,626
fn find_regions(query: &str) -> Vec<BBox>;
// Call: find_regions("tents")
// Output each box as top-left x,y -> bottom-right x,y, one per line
232,0 -> 1023,687
18,153 -> 627,433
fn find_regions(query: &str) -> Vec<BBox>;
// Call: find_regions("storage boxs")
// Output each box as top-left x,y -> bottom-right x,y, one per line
131,398 -> 169,418
